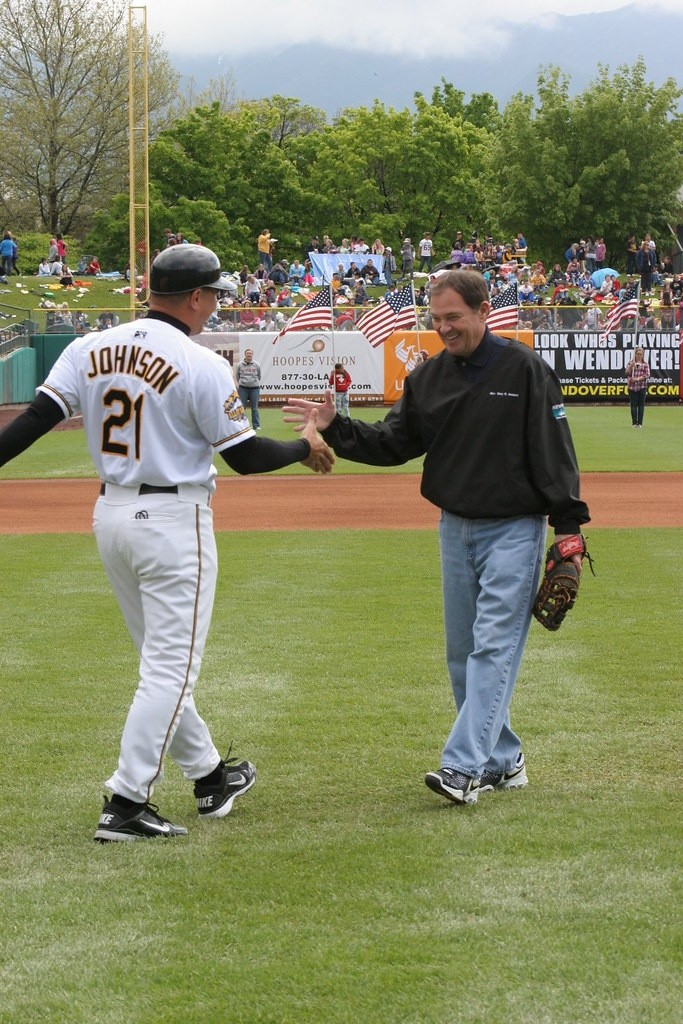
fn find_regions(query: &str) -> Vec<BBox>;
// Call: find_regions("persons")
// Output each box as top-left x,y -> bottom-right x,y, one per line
205,259 -> 314,333
282,270 -> 592,805
307,232 -> 683,331
0,244 -> 335,845
625,347 -> 650,428
39,233 -> 73,286
236,349 -> 262,430
44,302 -> 114,331
77,256 -> 101,275
0,234 -> 21,276
257,229 -> 272,270
124,258 -> 138,282
420,349 -> 429,361
150,228 -> 188,266
329,363 -> 352,417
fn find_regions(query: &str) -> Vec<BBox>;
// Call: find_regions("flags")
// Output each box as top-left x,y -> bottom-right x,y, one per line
272,285 -> 332,344
355,283 -> 416,348
485,284 -> 517,331
600,283 -> 639,344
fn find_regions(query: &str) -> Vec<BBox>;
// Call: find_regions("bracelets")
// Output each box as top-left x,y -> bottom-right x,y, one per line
637,378 -> 639,382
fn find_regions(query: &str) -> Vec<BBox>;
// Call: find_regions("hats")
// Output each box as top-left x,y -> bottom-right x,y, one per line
404,238 -> 411,244
385,246 -> 392,253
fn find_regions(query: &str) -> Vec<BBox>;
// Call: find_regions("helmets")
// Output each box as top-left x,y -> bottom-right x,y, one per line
149,244 -> 240,293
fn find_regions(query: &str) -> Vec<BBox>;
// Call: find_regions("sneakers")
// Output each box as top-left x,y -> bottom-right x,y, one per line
479,751 -> 530,792
425,766 -> 481,807
193,742 -> 257,818
92,793 -> 191,844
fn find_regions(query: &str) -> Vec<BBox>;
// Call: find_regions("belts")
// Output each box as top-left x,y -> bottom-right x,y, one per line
100,482 -> 179,495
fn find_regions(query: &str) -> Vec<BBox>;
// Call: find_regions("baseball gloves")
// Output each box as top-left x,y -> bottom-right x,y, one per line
531,533 -> 588,631
